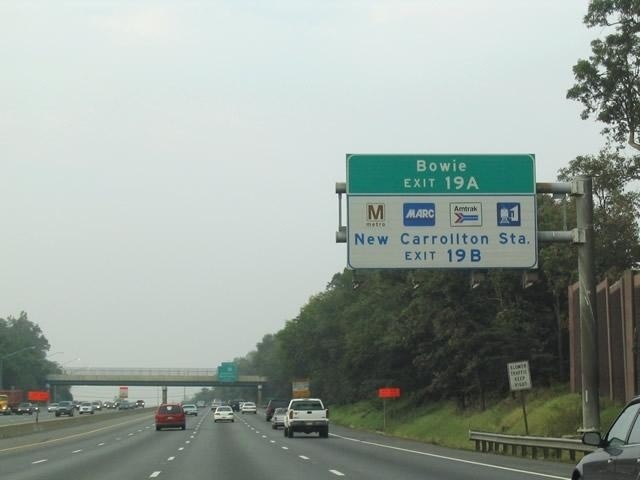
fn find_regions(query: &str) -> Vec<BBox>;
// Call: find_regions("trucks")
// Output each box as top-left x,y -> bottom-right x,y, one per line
0,389 -> 23,415
196,400 -> 205,407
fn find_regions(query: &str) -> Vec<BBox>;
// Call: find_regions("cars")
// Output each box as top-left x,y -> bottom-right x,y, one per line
569,392 -> 640,480
271,407 -> 288,430
152,401 -> 186,431
17,398 -> 147,416
209,399 -> 257,414
180,403 -> 200,417
212,405 -> 235,424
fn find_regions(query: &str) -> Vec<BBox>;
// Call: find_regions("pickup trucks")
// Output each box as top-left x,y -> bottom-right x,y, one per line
283,397 -> 330,439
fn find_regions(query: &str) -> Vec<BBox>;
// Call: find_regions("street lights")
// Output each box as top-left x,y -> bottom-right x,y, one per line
1,345 -> 37,363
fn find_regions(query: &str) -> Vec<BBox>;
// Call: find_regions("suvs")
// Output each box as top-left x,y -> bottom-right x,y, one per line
265,398 -> 290,422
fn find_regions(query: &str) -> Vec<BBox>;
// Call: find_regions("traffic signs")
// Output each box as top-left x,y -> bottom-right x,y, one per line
345,152 -> 536,195
222,362 -> 236,366
347,195 -> 539,271
217,366 -> 239,380
